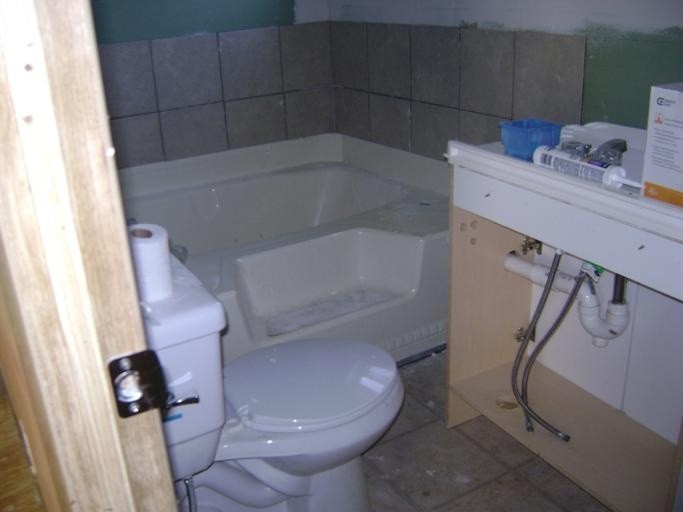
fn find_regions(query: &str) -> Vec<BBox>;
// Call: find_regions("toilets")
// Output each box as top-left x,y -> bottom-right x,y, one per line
141,254 -> 406,512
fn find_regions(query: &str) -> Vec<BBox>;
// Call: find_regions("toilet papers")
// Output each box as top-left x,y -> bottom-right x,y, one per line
128,222 -> 172,300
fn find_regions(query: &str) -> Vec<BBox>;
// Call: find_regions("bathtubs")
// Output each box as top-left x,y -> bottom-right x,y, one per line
123,162 -> 450,374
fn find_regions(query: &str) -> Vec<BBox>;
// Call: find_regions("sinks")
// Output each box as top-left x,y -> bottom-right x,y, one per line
561,120 -> 647,184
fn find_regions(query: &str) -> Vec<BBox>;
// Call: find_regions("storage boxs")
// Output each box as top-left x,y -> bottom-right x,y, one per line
639,82 -> 683,207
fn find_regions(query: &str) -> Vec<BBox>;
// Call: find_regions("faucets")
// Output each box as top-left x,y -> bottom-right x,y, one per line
586,138 -> 627,160
